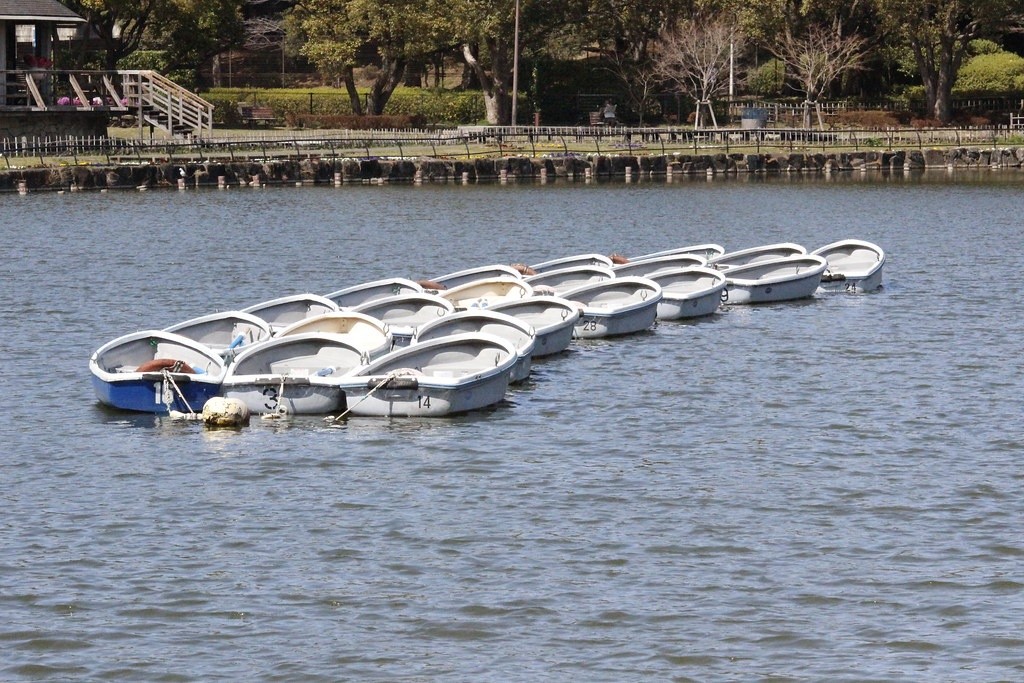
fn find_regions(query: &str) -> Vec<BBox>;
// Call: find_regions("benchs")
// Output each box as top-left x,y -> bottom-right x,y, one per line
662,285 -> 703,296
589,112 -> 625,128
269,356 -> 355,378
114,365 -> 194,373
587,299 -> 641,310
829,257 -> 875,273
241,106 -> 278,130
520,315 -> 558,329
421,363 -> 487,380
381,317 -> 431,328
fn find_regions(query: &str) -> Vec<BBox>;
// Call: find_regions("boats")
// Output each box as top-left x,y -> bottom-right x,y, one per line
557,275 -> 664,340
419,263 -> 522,304
509,240 -> 725,299
87,330 -> 228,413
273,310 -> 394,364
708,240 -> 808,285
237,292 -> 341,334
225,334 -> 369,415
342,331 -> 519,418
161,309 -> 272,364
348,291 -> 456,350
408,308 -> 536,386
437,276 -> 534,315
482,296 -> 580,360
718,254 -> 832,306
811,238 -> 888,294
326,275 -> 424,313
651,266 -> 728,321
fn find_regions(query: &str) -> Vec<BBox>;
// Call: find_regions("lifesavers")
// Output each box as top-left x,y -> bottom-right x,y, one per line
606,251 -> 631,265
509,261 -> 537,278
133,362 -> 200,377
414,282 -> 446,291
